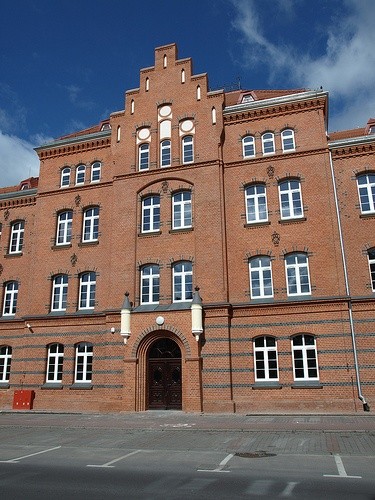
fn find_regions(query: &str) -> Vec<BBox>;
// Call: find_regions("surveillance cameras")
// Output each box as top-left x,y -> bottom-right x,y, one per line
27,323 -> 31,328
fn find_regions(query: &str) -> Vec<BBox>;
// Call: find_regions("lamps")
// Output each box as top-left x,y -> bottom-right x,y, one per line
191,286 -> 204,342
119,290 -> 132,345
